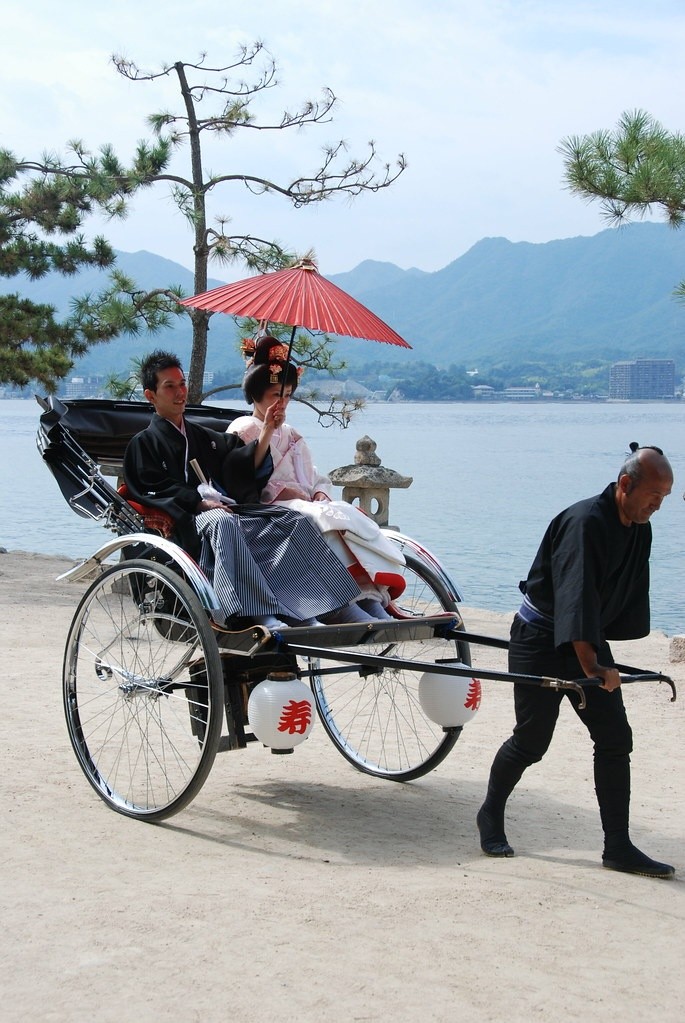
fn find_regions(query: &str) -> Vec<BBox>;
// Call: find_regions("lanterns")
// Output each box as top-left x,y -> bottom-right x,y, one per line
247,672 -> 317,755
418,657 -> 482,732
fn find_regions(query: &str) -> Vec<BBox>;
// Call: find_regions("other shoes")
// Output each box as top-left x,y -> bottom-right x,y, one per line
324,603 -> 378,624
285,616 -> 326,627
477,807 -> 514,857
358,598 -> 396,620
265,621 -> 290,631
602,841 -> 675,877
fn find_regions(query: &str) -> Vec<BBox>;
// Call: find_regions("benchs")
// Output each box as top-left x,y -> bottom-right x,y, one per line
113,479 -> 463,655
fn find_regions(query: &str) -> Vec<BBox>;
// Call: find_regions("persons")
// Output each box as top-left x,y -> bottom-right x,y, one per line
123,348 -> 361,629
225,338 -> 406,622
475,441 -> 677,877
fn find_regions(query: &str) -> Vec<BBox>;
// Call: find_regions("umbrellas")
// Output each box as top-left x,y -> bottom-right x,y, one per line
178,257 -> 412,428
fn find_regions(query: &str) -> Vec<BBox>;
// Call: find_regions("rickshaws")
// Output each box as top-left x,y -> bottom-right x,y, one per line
29,393 -> 679,826
